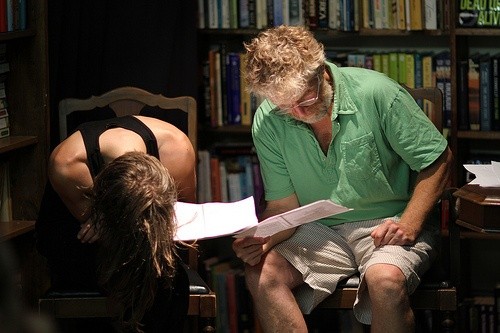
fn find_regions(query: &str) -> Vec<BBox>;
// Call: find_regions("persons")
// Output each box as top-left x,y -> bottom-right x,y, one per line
233,26 -> 455,333
33,113 -> 197,333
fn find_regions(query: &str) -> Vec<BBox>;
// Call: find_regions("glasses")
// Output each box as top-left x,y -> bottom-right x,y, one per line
266,73 -> 320,115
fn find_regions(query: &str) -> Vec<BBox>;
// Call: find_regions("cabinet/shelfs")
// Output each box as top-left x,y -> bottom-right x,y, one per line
0,0 -> 51,333
184,0 -> 500,333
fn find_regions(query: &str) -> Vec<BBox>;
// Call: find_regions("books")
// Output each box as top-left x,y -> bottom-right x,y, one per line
0,0 -> 500,274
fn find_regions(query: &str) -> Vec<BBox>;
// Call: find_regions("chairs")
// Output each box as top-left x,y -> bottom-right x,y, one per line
315,83 -> 459,333
36,86 -> 218,333
